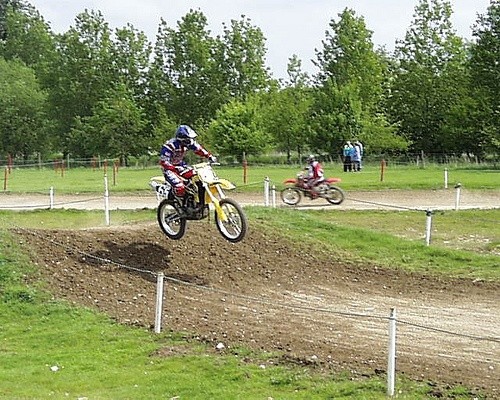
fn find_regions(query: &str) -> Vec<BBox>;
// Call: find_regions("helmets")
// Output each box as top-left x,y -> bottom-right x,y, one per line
176,125 -> 198,148
306,155 -> 315,164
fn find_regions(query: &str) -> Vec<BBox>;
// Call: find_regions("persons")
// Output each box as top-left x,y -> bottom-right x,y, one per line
161,124 -> 217,202
341,139 -> 362,172
296,154 -> 325,193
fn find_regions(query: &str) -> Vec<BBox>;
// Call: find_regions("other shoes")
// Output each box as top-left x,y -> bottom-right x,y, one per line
174,195 -> 187,207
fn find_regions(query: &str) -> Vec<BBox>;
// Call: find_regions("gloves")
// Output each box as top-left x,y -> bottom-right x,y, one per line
208,155 -> 217,163
175,166 -> 185,173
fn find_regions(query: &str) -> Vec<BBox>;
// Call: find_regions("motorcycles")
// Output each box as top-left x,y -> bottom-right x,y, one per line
149,160 -> 247,243
279,170 -> 345,205
